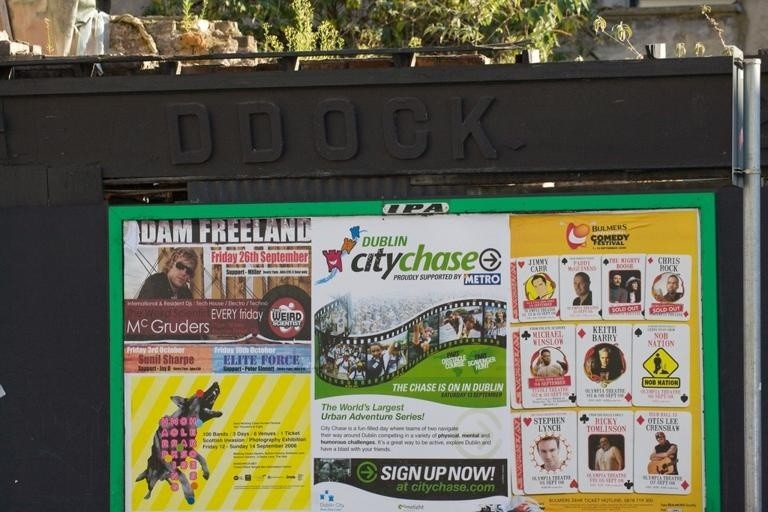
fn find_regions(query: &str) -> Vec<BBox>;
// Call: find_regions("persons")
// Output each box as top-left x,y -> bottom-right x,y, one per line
330,461 -> 346,482
315,462 -> 331,482
648,432 -> 678,474
662,274 -> 682,301
592,437 -> 622,471
570,271 -> 592,304
536,436 -> 561,470
608,273 -> 627,303
530,273 -> 550,300
624,276 -> 640,303
317,297 -> 506,382
135,246 -> 197,300
533,349 -> 563,375
596,347 -> 612,368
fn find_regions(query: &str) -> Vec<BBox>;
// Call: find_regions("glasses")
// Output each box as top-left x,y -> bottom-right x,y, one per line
174,261 -> 194,278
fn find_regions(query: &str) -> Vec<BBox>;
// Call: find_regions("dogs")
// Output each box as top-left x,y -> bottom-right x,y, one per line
134,381 -> 225,505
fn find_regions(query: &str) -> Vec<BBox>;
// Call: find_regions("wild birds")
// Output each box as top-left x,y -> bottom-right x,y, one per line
108,11 -> 158,58
